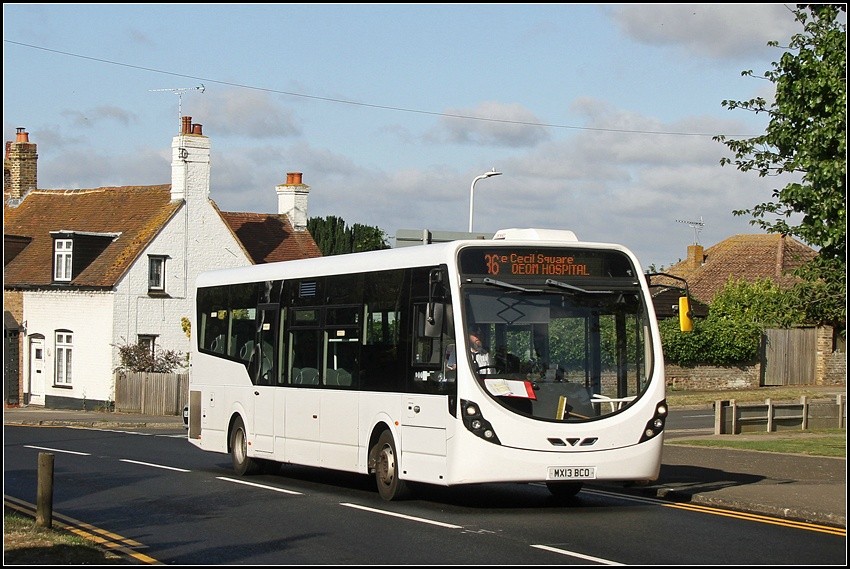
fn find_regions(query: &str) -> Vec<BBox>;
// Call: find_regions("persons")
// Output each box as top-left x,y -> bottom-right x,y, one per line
445,322 -> 498,380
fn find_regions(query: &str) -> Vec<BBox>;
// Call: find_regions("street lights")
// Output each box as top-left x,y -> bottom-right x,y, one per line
469,172 -> 504,233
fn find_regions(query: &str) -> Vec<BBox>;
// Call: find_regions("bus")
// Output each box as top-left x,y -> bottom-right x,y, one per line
187,227 -> 695,503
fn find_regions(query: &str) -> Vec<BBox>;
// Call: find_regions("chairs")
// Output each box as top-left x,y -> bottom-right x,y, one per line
209,334 -> 358,391
496,354 -> 520,373
442,343 -> 456,373
